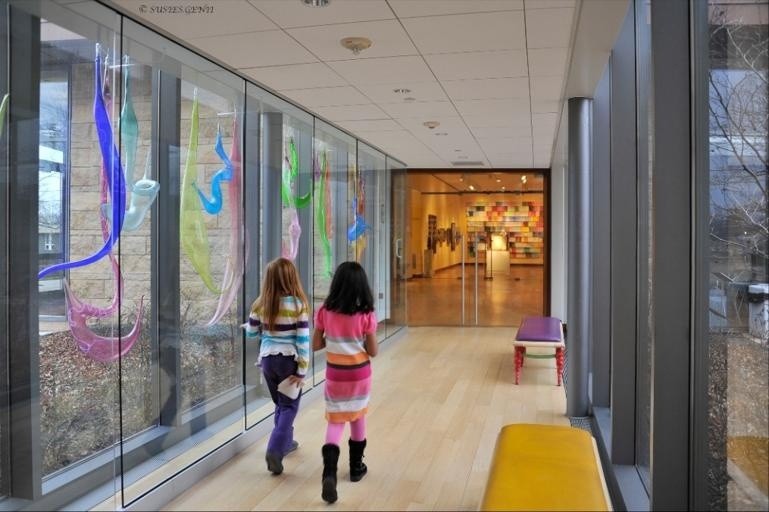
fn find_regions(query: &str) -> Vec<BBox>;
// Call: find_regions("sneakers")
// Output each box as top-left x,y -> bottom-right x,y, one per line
266,450 -> 283,474
282,441 -> 298,456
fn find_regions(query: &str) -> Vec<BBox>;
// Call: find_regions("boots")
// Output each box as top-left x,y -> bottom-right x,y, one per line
322,444 -> 340,503
348,438 -> 367,482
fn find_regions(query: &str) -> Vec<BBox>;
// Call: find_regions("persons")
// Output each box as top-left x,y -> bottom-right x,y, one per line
312,260 -> 378,503
245,258 -> 310,476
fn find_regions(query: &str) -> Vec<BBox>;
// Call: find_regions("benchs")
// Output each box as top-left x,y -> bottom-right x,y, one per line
477,417 -> 617,512
507,313 -> 567,387
